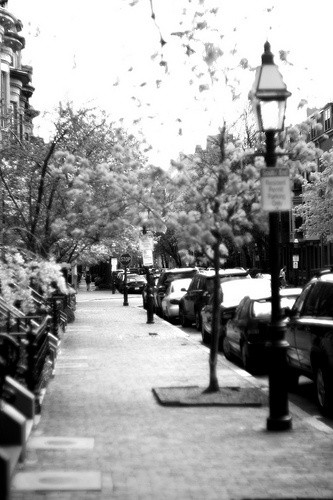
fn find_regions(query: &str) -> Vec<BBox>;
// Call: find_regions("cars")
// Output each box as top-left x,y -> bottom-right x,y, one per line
221,287 -> 304,377
199,272 -> 272,353
112,266 -> 222,315
157,277 -> 192,324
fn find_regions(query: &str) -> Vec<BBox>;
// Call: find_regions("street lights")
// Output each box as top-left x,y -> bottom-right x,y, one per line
246,40 -> 296,434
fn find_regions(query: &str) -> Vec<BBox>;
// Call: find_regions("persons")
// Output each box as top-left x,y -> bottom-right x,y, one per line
85,271 -> 92,291
280,266 -> 288,288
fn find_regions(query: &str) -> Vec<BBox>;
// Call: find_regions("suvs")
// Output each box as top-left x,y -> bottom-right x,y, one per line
178,266 -> 252,331
283,268 -> 333,418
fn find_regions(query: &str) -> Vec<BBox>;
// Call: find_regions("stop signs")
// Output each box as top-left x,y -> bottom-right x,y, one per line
119,254 -> 132,266
